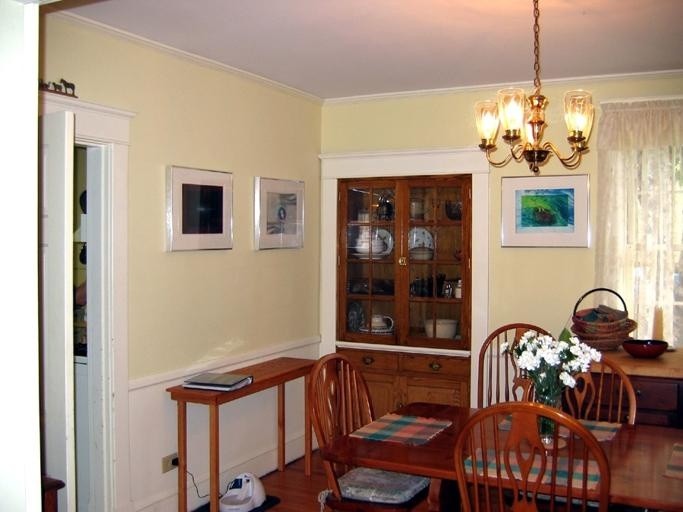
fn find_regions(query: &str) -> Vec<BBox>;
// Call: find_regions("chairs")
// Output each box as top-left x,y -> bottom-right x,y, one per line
477,323 -> 558,409
305,352 -> 431,511
554,353 -> 636,426
452,401 -> 612,511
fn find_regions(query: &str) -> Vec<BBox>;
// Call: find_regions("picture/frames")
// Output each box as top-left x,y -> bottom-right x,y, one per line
501,174 -> 588,247
255,177 -> 306,249
166,165 -> 235,251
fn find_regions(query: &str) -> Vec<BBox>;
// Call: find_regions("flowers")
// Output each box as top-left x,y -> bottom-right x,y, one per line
499,328 -> 602,388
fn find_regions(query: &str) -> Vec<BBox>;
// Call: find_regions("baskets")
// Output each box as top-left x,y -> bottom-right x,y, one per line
570,289 -> 638,352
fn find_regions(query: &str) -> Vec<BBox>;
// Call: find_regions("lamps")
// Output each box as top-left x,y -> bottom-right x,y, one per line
475,1 -> 595,171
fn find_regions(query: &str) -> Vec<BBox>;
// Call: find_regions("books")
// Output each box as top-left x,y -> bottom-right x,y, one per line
182,373 -> 253,392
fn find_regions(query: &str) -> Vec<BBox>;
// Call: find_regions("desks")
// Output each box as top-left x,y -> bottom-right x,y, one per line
167,357 -> 317,512
320,401 -> 683,512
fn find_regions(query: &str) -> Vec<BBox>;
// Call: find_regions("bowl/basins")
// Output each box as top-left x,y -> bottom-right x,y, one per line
622,340 -> 668,359
424,318 -> 459,338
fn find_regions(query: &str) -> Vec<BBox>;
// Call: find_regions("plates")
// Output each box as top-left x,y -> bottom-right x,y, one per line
408,226 -> 435,252
351,228 -> 394,260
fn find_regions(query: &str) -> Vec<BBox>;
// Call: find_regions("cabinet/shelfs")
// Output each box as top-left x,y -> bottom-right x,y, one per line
562,348 -> 683,426
315,145 -> 490,418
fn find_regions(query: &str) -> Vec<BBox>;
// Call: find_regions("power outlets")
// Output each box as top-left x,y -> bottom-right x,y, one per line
162,453 -> 182,473
